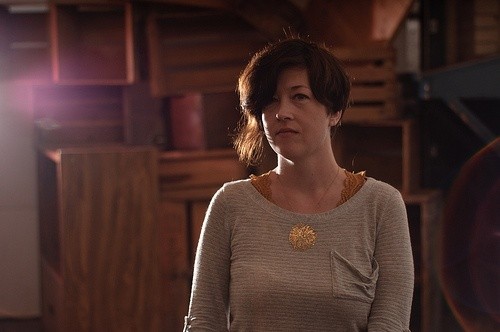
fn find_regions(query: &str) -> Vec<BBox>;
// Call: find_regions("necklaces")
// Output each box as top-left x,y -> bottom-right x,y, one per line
266,164 -> 341,252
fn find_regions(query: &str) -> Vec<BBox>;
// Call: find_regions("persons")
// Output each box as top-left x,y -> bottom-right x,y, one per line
181,36 -> 415,331
